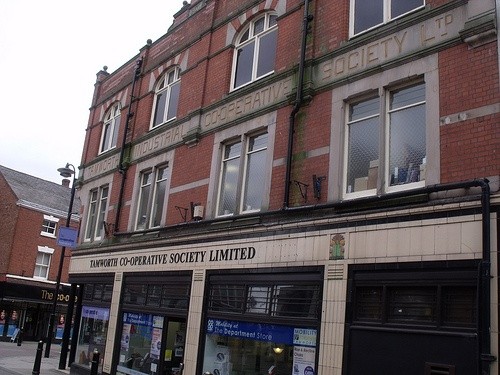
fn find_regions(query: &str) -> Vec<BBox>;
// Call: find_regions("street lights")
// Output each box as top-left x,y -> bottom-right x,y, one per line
44,163 -> 82,360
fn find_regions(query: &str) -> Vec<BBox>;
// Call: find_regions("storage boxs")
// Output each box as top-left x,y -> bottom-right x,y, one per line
353,158 -> 379,192
